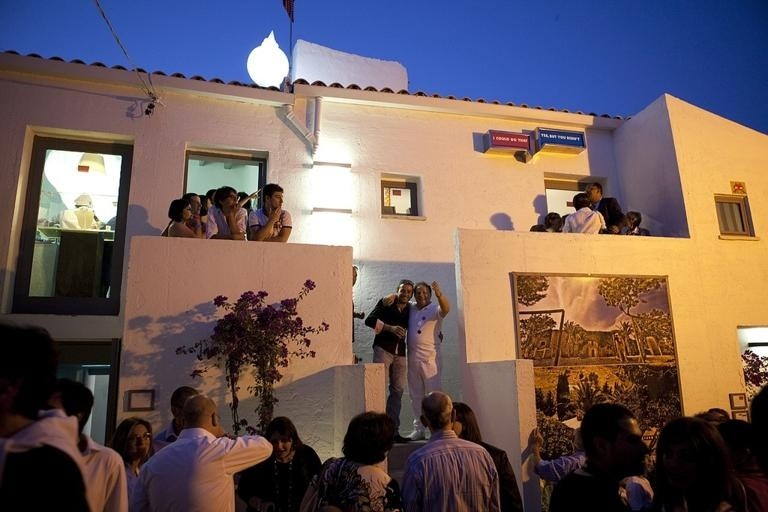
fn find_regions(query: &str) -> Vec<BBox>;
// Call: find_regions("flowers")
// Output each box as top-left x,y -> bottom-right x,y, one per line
741,347 -> 767,394
180,278 -> 329,427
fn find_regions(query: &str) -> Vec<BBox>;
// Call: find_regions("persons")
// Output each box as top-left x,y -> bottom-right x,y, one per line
363,279 -> 444,443
113,383 -> 768,510
93,212 -> 118,297
44,194 -> 106,231
381,280 -> 452,441
2,324 -> 128,511
161,182 -> 293,243
530,181 -> 653,238
351,264 -> 367,365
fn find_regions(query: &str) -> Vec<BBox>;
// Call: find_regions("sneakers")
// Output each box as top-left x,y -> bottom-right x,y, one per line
393,433 -> 411,444
406,431 -> 425,441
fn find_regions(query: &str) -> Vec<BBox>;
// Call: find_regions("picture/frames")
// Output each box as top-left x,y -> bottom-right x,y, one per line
128,389 -> 156,411
729,392 -> 749,422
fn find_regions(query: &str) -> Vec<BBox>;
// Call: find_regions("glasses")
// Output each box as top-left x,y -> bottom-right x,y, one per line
229,195 -> 240,201
129,433 -> 151,441
187,208 -> 194,212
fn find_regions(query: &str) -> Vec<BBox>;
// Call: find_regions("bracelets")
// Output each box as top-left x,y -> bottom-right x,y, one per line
437,291 -> 442,298
